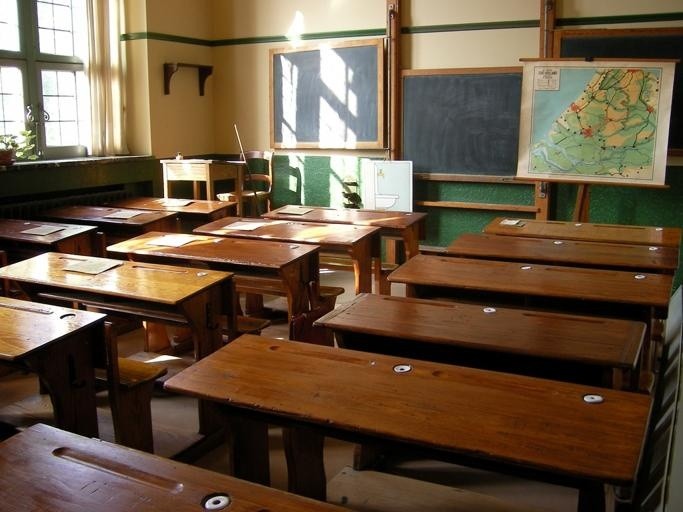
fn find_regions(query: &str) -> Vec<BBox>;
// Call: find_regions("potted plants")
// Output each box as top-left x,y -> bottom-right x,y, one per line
0,129 -> 40,165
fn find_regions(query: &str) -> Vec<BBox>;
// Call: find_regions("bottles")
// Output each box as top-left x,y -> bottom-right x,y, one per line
175,152 -> 183,160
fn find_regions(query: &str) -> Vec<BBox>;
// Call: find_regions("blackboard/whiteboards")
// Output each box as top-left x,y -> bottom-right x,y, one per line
555,27 -> 683,156
399,66 -> 522,184
268,38 -> 384,149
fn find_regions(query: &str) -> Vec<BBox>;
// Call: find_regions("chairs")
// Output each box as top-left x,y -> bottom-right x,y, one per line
216,150 -> 274,217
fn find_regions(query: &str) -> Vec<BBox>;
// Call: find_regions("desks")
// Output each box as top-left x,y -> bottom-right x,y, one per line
159,159 -> 246,217
